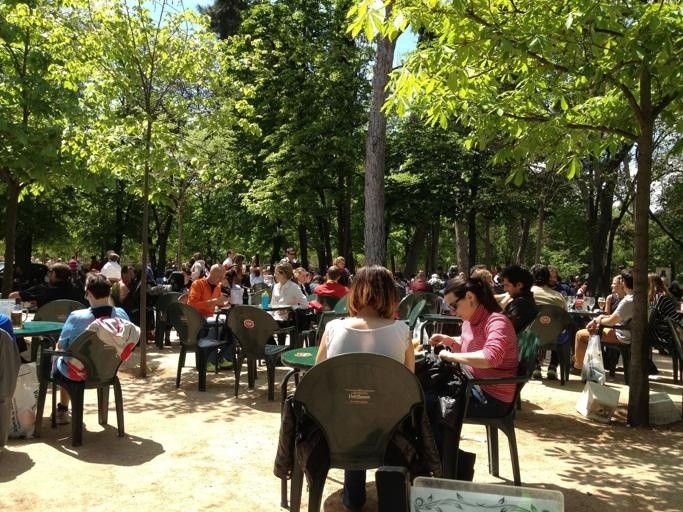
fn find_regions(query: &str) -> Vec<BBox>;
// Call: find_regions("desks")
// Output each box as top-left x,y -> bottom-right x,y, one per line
0,319 -> 71,428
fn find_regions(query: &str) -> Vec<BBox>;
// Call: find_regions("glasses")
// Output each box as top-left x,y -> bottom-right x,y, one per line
448,297 -> 463,311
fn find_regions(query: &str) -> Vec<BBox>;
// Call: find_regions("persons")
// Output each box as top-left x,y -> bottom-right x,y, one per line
0,249 -> 142,446
394,265 -> 503,295
315,265 -> 415,511
501,264 -> 683,380
426,274 -> 518,482
146,249 -> 354,371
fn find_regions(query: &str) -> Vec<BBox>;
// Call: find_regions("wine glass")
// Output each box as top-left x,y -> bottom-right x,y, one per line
566,295 -> 606,312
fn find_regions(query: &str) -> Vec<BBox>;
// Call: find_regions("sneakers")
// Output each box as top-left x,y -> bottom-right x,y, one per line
547,365 -> 557,380
50,408 -> 70,424
218,359 -> 233,370
532,370 -> 542,379
568,367 -> 581,375
647,366 -> 658,376
459,450 -> 476,482
206,362 -> 220,371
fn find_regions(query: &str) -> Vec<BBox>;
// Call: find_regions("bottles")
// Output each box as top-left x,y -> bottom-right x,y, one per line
261,290 -> 269,308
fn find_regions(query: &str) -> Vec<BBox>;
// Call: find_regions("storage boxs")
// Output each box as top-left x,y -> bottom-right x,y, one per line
408,472 -> 565,511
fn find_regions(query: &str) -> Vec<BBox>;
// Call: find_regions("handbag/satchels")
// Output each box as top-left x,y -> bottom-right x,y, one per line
420,362 -> 465,433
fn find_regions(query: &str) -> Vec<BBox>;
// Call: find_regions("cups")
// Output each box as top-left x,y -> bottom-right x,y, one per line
11,304 -> 28,330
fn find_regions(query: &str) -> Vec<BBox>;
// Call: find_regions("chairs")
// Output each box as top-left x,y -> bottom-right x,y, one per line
1,326 -> 23,450
34,316 -> 143,448
144,262 -> 351,400
32,299 -> 88,428
448,328 -> 541,497
389,275 -> 683,411
